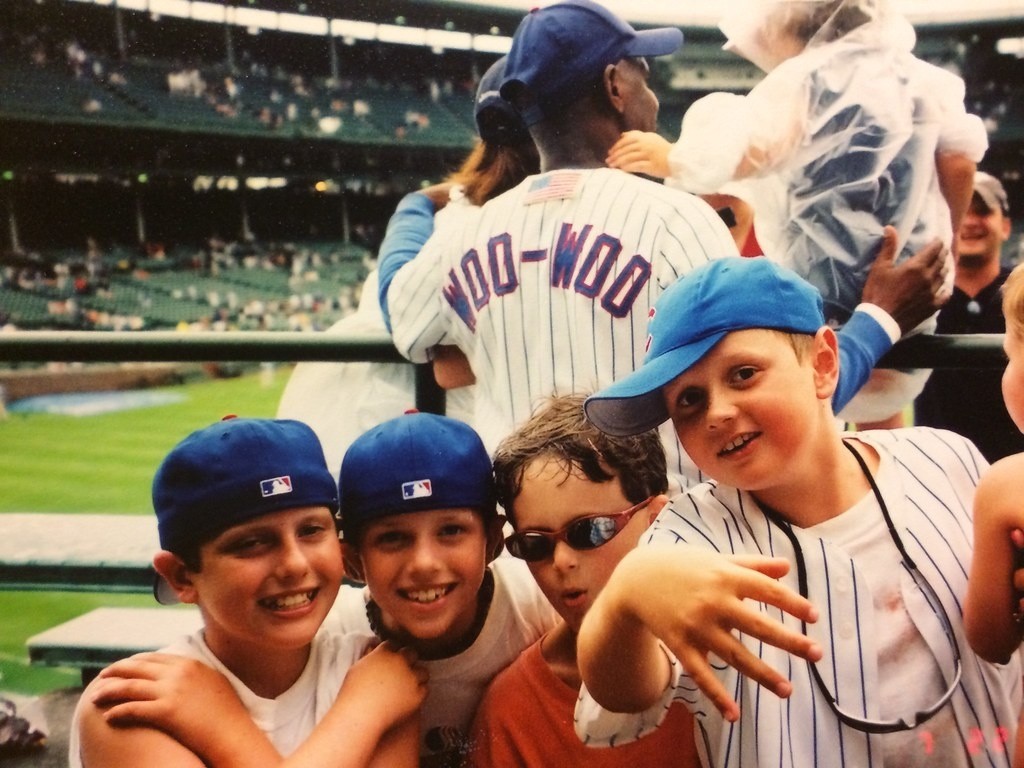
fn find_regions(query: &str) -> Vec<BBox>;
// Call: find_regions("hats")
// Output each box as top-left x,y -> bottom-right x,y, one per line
339,409 -> 497,529
583,255 -> 825,439
475,56 -> 526,138
151,413 -> 338,606
499,0 -> 685,128
972,171 -> 1009,213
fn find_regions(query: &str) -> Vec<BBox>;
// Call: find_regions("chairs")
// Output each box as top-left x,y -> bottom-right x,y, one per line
0,48 -> 474,335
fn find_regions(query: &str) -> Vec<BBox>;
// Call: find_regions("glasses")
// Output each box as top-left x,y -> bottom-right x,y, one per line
505,495 -> 658,563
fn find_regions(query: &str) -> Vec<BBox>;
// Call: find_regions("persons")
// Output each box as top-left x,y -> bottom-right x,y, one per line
73,0 -> 1024,766
